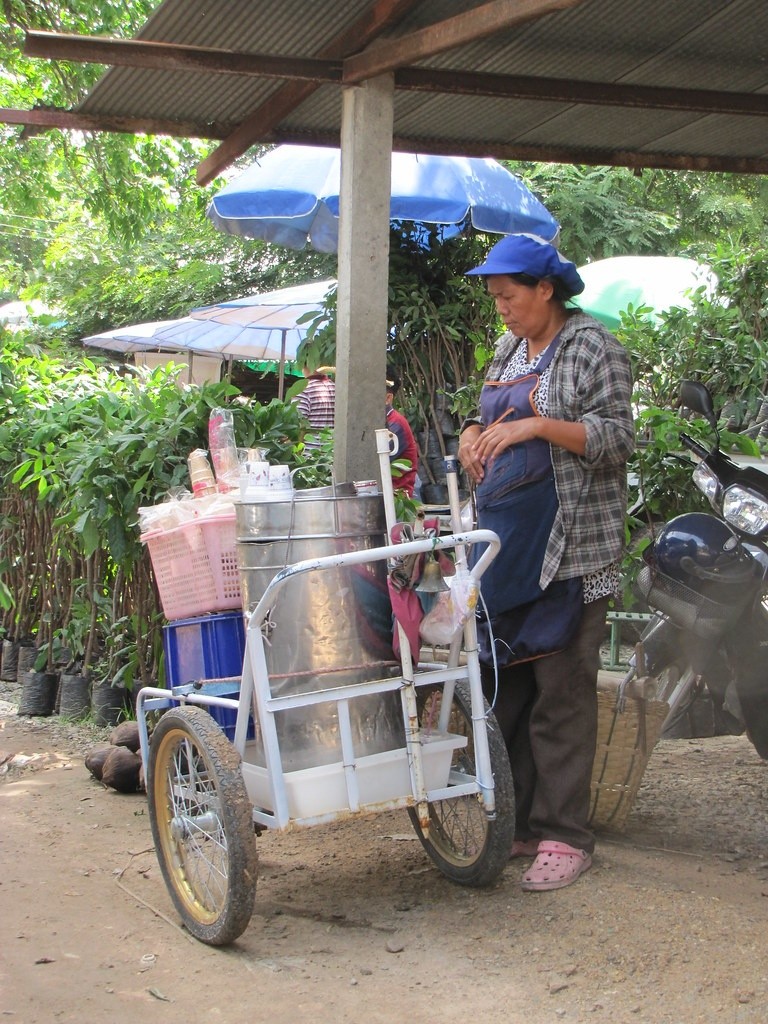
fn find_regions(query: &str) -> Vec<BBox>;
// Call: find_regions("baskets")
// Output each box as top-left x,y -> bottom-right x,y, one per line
630,537 -> 766,643
140,513 -> 244,620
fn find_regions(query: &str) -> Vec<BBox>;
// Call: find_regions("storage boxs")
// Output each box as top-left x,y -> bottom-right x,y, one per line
139,516 -> 256,742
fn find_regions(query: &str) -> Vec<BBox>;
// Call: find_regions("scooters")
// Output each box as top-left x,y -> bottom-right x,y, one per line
573,380 -> 768,834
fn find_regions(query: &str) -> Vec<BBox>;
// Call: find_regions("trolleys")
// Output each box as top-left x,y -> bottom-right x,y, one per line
135,528 -> 515,947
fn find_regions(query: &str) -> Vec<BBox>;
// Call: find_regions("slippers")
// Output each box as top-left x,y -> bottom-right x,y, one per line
507,840 -> 540,859
522,839 -> 592,889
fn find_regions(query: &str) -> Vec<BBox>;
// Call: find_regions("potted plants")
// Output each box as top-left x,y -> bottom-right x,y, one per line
0,341 -> 299,727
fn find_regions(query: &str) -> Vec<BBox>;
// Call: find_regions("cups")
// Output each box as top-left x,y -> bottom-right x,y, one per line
244,462 -> 293,501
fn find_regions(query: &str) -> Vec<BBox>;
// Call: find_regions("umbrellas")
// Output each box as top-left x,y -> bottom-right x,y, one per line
76,278 -> 427,403
207,142 -> 559,255
564,255 -> 733,335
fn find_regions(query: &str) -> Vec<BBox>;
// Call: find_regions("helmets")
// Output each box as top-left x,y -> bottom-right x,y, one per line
650,512 -> 762,607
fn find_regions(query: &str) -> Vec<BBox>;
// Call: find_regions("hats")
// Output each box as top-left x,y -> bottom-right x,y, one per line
463,232 -> 587,301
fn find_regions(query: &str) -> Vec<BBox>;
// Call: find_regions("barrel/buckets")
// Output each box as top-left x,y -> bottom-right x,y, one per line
232,464 -> 407,773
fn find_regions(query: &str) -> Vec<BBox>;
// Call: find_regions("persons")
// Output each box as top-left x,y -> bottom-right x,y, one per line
385,364 -> 418,499
291,342 -> 335,458
456,234 -> 636,890
